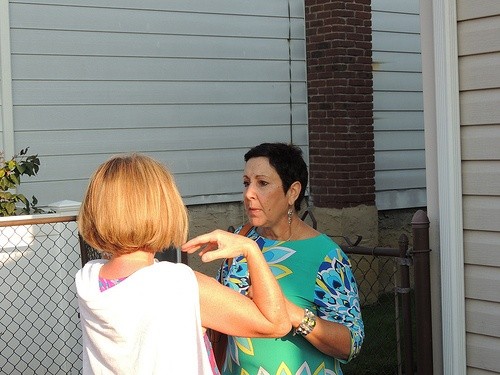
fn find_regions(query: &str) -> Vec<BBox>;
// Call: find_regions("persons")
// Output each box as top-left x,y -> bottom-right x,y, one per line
73,153 -> 293,375
208,141 -> 365,375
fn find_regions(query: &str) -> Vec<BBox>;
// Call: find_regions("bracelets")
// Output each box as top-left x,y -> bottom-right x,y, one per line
294,308 -> 316,337
